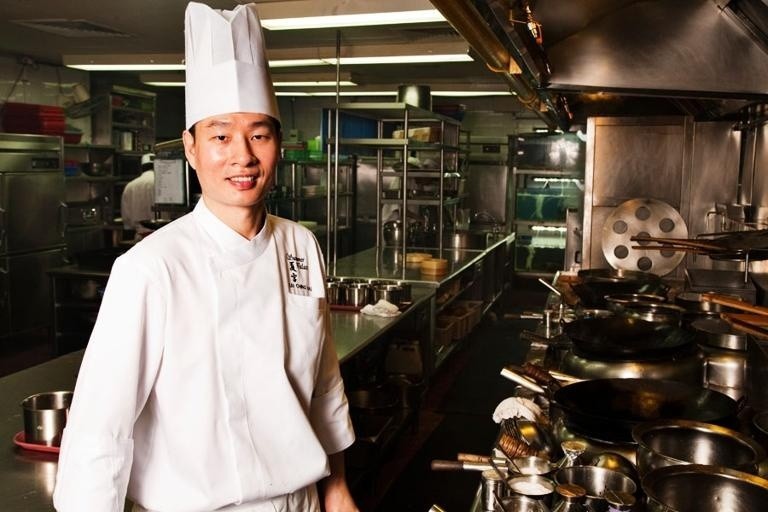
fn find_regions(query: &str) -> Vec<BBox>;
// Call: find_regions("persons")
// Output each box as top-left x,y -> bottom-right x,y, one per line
52,1 -> 360,511
120,152 -> 171,241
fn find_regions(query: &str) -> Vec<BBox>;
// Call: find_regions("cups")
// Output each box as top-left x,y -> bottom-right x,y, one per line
401,283 -> 412,305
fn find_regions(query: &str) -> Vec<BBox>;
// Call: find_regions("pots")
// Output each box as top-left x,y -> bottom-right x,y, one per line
139,219 -> 171,230
382,221 -> 404,245
517,269 -> 749,447
629,419 -> 768,511
432,448 -> 636,512
398,84 -> 432,111
67,277 -> 105,302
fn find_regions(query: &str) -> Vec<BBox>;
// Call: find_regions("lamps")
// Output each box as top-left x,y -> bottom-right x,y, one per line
267,46 -> 330,68
63,53 -> 185,72
276,81 -> 404,96
139,73 -> 186,87
431,84 -> 513,97
320,34 -> 477,66
271,73 -> 361,86
256,0 -> 447,30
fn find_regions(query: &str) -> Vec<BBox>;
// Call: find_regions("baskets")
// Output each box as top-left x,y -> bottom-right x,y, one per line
438,310 -> 473,340
447,301 -> 483,329
434,319 -> 456,345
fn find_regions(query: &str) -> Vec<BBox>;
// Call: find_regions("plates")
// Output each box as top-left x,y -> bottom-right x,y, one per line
13,428 -> 59,454
398,252 -> 448,270
348,384 -> 397,410
302,185 -> 325,198
385,372 -> 424,408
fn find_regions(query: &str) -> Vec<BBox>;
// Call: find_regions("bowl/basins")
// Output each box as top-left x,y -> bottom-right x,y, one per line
82,162 -> 112,176
328,276 -> 401,305
20,390 -> 74,447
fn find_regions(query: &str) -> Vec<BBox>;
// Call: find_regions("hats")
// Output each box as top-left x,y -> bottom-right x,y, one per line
140,153 -> 155,165
183,0 -> 282,130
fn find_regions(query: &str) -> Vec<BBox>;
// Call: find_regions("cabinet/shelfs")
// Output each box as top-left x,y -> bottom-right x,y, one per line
91,88 -> 156,225
62,143 -> 116,226
326,102 -> 462,279
274,160 -> 353,233
46,266 -> 109,357
339,291 -> 438,470
435,231 -> 518,368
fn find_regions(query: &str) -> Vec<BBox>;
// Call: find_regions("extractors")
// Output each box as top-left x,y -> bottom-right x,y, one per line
423,0 -> 768,133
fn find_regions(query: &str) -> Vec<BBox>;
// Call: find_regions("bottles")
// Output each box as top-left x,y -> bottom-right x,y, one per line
120,130 -> 136,151
551,484 -> 636,512
